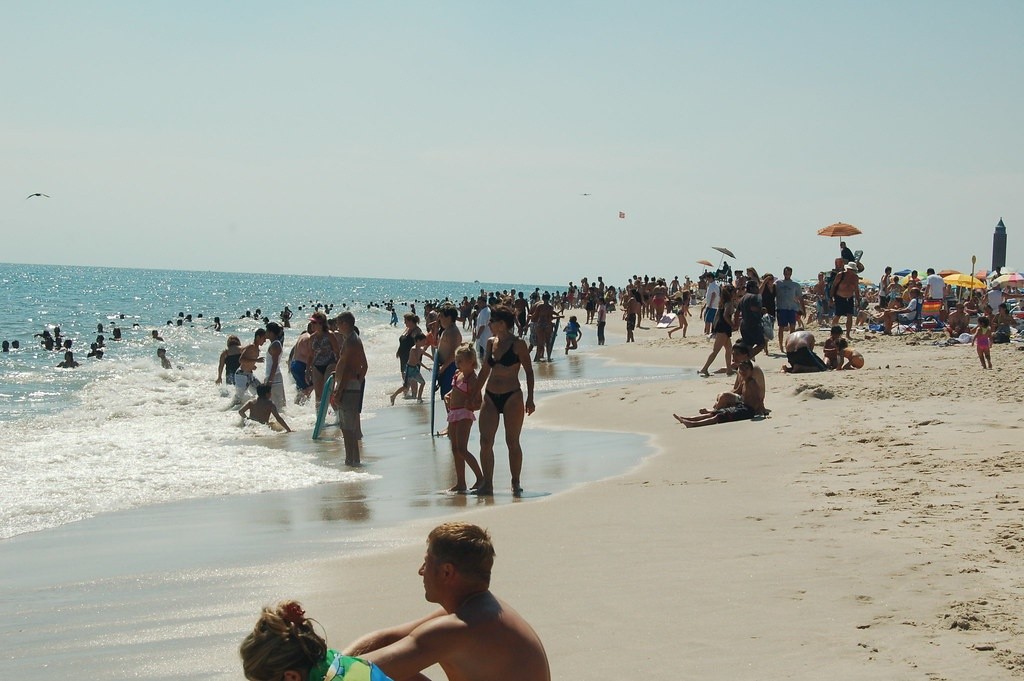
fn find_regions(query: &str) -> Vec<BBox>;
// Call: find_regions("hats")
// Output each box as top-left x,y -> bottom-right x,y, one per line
844,261 -> 858,270
308,317 -> 322,322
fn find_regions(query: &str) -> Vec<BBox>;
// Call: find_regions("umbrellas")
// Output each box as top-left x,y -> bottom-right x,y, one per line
697,258 -> 713,274
817,220 -> 862,253
711,245 -> 735,272
798,269 -> 1024,306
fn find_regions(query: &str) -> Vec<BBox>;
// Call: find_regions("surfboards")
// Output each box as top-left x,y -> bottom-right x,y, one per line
428,348 -> 440,437
311,374 -> 335,439
551,318 -> 559,354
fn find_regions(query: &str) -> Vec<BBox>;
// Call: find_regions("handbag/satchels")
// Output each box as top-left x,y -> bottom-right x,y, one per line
761,312 -> 774,339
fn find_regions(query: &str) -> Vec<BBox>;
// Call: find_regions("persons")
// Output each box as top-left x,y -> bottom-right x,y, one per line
367,277 -> 618,406
972,316 -> 993,369
673,342 -> 766,428
440,344 -> 483,492
617,241 -> 881,376
879,266 -> 944,334
205,300 -> 368,465
240,599 -> 394,681
3,308 -> 203,371
342,524 -> 551,681
946,281 -> 1024,340
471,310 -> 535,495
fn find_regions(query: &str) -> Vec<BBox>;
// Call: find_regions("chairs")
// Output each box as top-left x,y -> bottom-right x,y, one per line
896,297 -> 942,335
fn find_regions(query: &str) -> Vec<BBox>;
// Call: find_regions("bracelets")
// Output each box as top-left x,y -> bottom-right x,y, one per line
473,401 -> 478,409
305,369 -> 313,374
